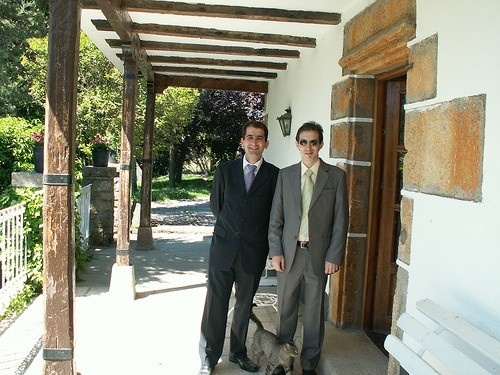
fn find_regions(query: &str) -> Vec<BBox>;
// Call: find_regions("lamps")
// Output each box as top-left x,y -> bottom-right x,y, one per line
276,107 -> 292,138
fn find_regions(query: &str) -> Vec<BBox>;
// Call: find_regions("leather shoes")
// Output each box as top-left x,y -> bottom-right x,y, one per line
229,355 -> 258,373
199,366 -> 213,375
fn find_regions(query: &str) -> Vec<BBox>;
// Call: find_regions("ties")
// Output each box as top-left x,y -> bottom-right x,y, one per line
244,164 -> 258,194
299,170 -> 315,237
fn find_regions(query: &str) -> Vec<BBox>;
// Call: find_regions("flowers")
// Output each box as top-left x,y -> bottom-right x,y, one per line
91,134 -> 112,149
31,127 -> 45,147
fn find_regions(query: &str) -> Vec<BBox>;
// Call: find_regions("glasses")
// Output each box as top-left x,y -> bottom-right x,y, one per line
298,139 -> 322,146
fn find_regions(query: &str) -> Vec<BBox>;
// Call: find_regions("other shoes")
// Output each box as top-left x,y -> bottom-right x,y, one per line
272,365 -> 294,375
302,369 -> 316,375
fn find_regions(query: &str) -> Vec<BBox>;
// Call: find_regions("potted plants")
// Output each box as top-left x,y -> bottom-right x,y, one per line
31,127 -> 44,144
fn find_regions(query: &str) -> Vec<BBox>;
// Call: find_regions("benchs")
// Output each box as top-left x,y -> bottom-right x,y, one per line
382,299 -> 500,375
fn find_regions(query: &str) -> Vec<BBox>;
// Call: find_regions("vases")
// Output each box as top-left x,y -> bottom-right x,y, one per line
91,150 -> 109,167
33,147 -> 43,173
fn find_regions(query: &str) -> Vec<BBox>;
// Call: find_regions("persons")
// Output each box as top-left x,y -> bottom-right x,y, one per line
268,121 -> 348,375
198,121 -> 280,375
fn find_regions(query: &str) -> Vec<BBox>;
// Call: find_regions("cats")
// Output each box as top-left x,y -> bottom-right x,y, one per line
248,303 -> 300,375
